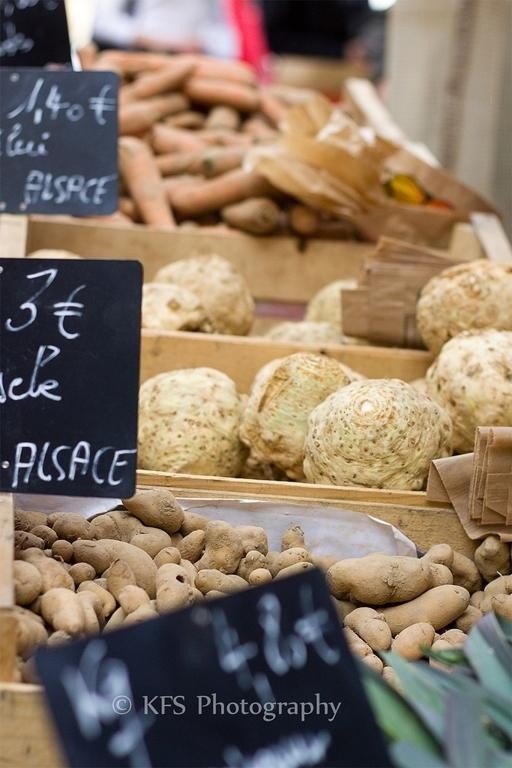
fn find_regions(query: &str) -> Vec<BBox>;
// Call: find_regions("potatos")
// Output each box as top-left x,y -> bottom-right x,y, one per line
2,488 -> 512,691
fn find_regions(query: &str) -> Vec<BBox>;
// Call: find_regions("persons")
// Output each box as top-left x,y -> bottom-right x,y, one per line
94,0 -> 242,57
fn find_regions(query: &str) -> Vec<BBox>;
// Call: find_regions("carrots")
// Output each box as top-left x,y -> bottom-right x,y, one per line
87,50 -> 352,238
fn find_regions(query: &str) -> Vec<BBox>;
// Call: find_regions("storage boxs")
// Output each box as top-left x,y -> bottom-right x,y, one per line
0,77 -> 511,768
